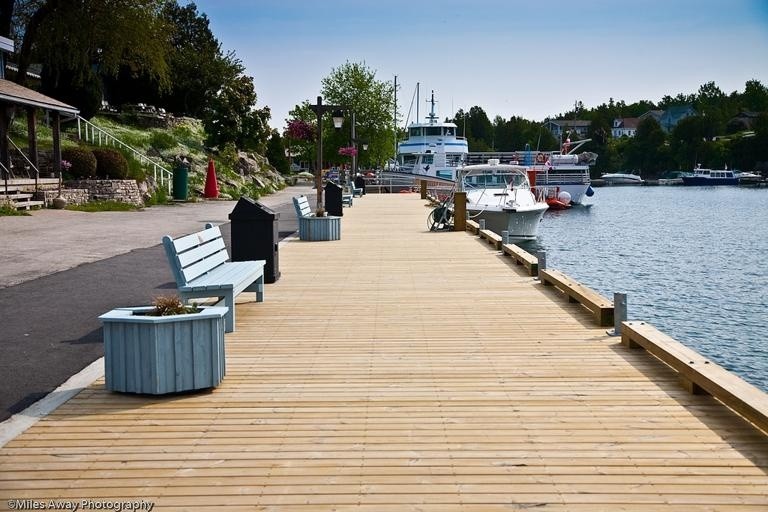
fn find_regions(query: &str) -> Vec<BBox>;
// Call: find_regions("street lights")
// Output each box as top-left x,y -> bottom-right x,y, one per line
348,134 -> 369,186
308,96 -> 349,214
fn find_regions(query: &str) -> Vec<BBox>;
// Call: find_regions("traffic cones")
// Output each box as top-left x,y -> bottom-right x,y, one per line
204,158 -> 219,198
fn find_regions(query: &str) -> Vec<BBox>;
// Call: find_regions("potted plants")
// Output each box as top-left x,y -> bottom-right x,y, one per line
97,294 -> 230,395
299,207 -> 342,240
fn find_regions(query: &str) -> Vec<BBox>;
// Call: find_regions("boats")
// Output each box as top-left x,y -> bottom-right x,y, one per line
735,172 -> 761,185
680,163 -> 738,186
602,172 -> 644,185
380,72 -> 599,243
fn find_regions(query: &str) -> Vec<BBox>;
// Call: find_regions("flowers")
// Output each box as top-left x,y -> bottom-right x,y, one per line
337,146 -> 358,157
280,116 -> 316,142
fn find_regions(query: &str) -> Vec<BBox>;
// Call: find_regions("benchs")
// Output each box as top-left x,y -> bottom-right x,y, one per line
337,181 -> 363,207
162,223 -> 267,333
292,195 -> 328,234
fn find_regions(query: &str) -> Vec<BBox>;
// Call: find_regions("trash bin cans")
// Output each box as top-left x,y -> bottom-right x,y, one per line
421,180 -> 427,199
229,193 -> 280,283
173,167 -> 188,200
355,176 -> 365,195
324,181 -> 343,216
454,192 -> 466,231
